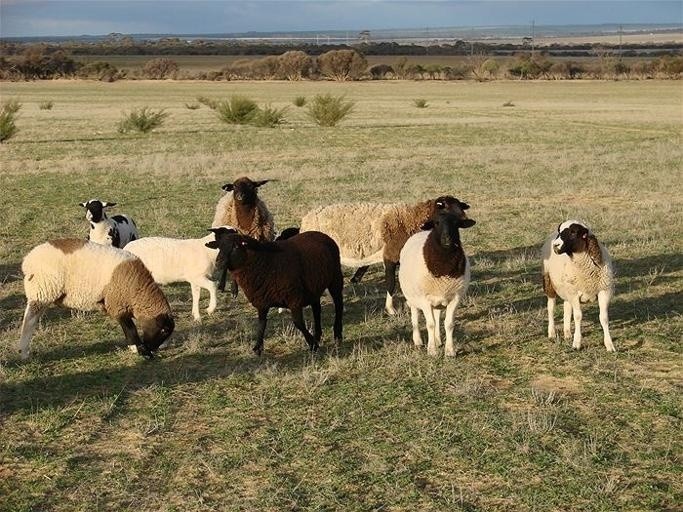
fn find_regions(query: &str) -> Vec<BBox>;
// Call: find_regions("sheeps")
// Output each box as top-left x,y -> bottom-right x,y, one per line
16,238 -> 175,361
540,219 -> 617,354
122,225 -> 239,324
398,208 -> 477,358
78,198 -> 138,250
298,195 -> 470,318
205,230 -> 344,357
211,176 -> 274,299
